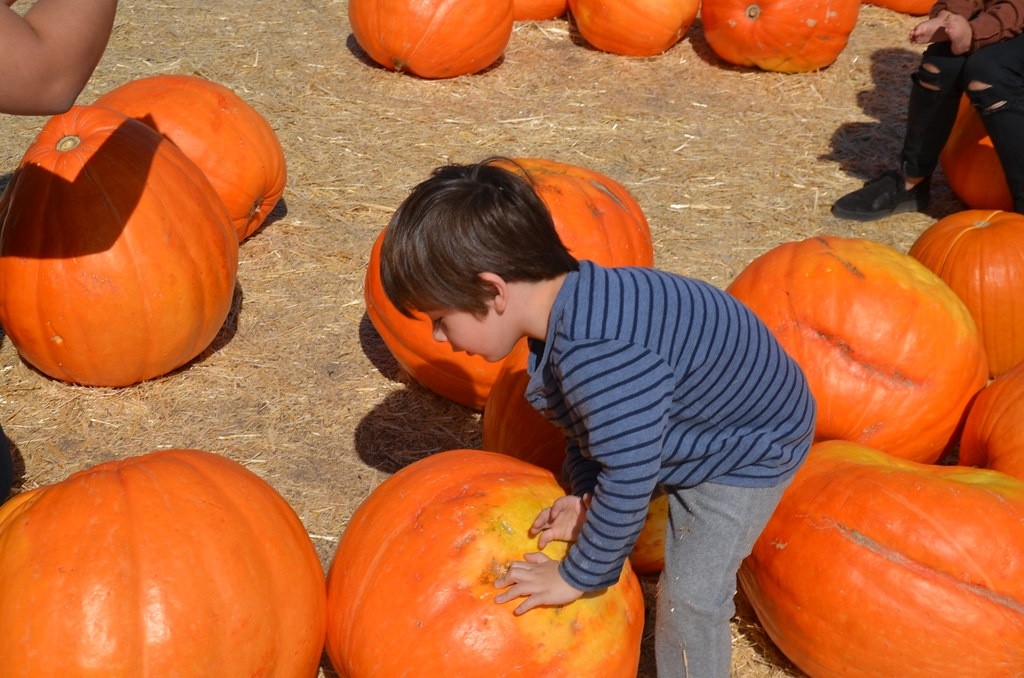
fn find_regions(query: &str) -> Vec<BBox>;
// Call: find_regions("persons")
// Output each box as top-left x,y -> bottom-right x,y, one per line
377,155 -> 816,678
831,0 -> 1024,220
0,0 -> 118,116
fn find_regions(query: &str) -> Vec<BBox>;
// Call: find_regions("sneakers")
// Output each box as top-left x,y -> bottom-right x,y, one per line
830,171 -> 932,222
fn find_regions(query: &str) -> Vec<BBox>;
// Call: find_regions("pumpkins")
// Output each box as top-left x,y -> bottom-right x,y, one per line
348,0 -> 938,81
0,69 -> 1024,678
